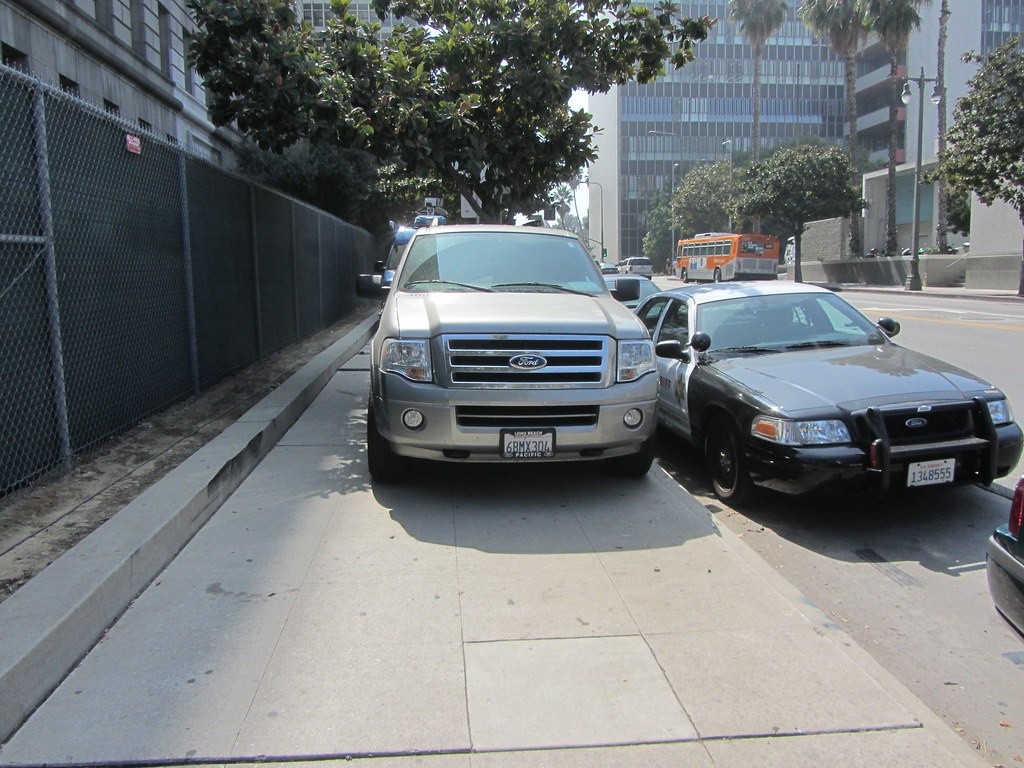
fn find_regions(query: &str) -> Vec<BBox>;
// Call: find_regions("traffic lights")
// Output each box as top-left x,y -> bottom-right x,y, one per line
603,249 -> 607,257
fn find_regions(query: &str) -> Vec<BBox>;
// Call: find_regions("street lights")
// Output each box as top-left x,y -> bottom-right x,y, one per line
722,140 -> 732,233
672,163 -> 680,275
647,130 -> 684,191
901,65 -> 941,291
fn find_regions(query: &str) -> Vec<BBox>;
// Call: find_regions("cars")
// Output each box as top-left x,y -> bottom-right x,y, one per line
368,215 -> 661,482
635,280 -> 1022,508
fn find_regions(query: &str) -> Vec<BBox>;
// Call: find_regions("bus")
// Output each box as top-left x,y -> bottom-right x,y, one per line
675,233 -> 779,283
